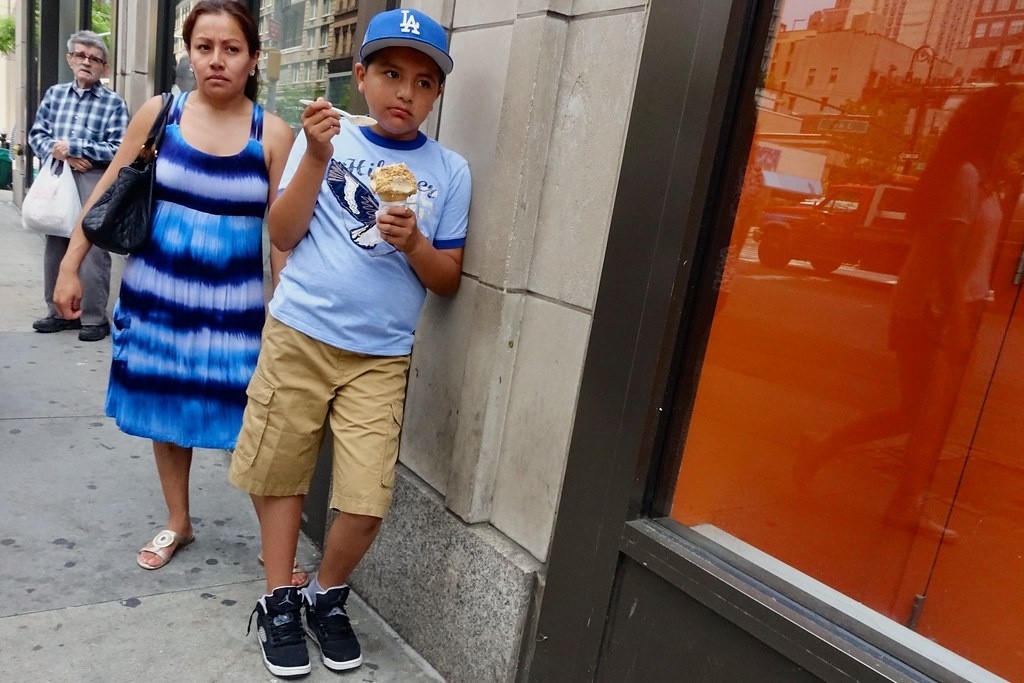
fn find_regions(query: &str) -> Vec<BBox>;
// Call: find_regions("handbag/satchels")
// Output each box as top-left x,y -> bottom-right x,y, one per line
81,93 -> 174,256
20,153 -> 83,238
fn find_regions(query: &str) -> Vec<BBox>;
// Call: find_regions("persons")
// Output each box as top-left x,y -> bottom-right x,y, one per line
790,85 -> 1023,541
28,34 -> 130,341
53,1 -> 309,589
229,8 -> 472,676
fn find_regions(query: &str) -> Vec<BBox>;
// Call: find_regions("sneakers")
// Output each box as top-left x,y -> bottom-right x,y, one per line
245,585 -> 312,676
33,316 -> 83,331
300,584 -> 363,671
79,320 -> 111,341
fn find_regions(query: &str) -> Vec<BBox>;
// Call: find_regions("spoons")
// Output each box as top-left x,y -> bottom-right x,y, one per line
299,99 -> 378,127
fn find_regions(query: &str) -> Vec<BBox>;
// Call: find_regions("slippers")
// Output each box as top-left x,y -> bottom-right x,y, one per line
259,551 -> 310,588
136,530 -> 196,569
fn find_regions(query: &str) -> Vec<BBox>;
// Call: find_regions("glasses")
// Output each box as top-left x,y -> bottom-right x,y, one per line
69,51 -> 106,66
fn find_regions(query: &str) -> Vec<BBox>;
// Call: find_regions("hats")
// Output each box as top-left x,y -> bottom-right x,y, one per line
358,8 -> 453,87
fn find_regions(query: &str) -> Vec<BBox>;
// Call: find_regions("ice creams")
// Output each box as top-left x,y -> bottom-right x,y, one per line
369,161 -> 418,202
